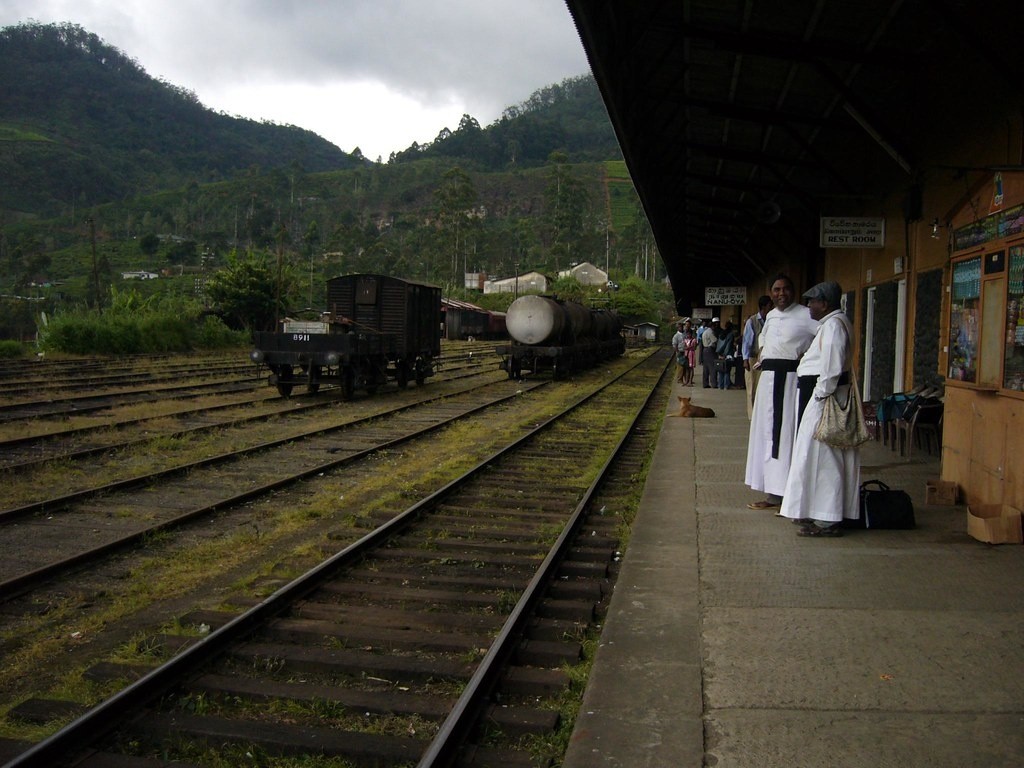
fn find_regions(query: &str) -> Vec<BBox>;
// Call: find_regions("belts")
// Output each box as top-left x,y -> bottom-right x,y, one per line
705,346 -> 713,348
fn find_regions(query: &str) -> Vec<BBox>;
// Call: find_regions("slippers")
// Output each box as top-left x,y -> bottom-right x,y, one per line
747,501 -> 780,510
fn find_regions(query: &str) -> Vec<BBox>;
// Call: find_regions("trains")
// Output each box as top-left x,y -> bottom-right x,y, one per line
495,294 -> 627,378
251,270 -> 445,396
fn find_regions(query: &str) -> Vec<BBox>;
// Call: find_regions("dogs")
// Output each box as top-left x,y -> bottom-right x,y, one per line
667,395 -> 715,418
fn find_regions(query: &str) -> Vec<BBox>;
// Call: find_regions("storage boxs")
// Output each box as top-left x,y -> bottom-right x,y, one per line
966,504 -> 1023,544
925,479 -> 958,505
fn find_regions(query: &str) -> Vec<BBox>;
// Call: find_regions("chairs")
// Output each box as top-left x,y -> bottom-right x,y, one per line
876,384 -> 951,465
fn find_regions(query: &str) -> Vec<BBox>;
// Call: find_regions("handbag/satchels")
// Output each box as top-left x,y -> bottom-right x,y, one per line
811,366 -> 874,448
677,356 -> 690,367
877,393 -> 918,422
902,396 -> 942,423
713,358 -> 730,373
856,479 -> 915,529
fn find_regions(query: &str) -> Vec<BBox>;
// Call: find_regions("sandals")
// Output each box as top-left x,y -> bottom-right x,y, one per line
792,519 -> 813,526
797,522 -> 844,538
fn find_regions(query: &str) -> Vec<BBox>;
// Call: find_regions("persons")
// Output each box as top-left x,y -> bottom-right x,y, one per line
741,293 -> 776,423
743,273 -> 822,517
672,317 -> 751,391
778,280 -> 863,539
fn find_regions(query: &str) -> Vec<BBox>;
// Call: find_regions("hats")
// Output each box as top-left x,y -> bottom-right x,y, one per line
803,281 -> 842,308
712,317 -> 719,322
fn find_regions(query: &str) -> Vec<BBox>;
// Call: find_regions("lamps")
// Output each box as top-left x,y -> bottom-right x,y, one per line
928,216 -> 945,239
754,200 -> 783,225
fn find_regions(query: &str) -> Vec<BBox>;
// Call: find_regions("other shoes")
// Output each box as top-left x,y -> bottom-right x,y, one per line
681,383 -> 693,387
703,385 -> 717,388
717,384 -> 738,390
